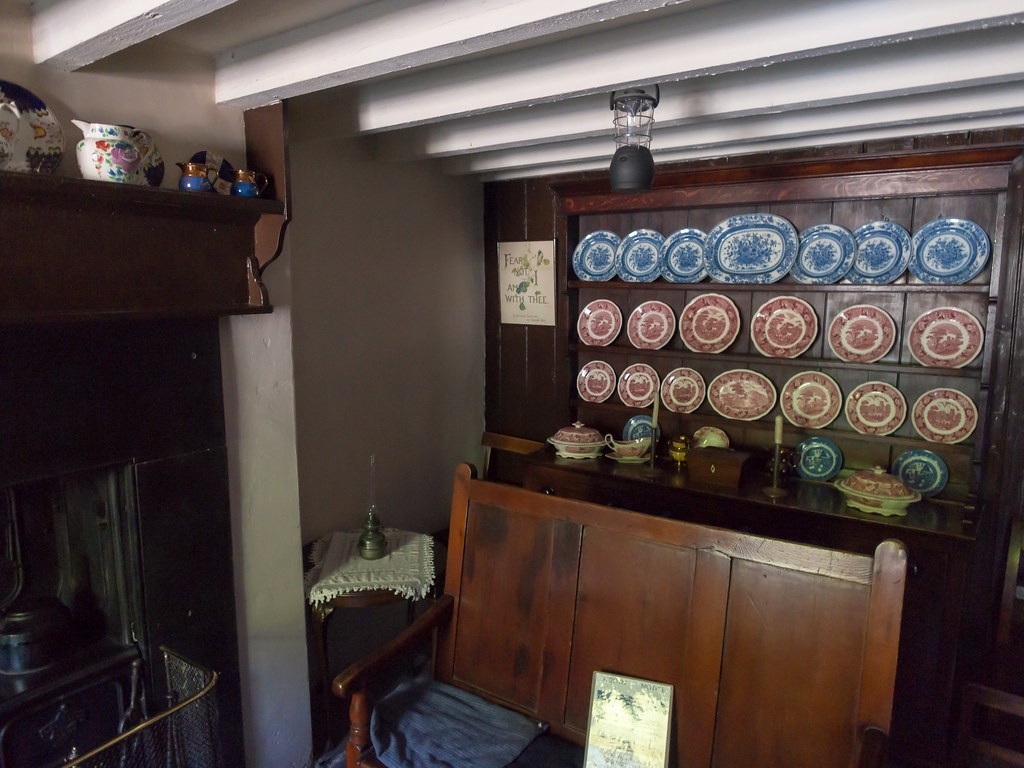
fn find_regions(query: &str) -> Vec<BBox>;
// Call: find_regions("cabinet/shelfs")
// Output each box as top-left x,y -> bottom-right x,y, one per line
517,141 -> 1024,768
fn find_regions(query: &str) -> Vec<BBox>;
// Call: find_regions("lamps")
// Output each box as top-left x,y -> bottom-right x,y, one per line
609,84 -> 661,196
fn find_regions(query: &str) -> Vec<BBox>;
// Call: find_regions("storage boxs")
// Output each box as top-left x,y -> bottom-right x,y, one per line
681,446 -> 753,490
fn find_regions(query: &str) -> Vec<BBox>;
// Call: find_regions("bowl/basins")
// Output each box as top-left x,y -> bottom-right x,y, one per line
834,465 -> 922,510
546,421 -> 611,453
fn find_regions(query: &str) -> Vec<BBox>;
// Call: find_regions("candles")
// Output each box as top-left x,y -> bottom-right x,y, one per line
774,413 -> 783,444
650,390 -> 659,430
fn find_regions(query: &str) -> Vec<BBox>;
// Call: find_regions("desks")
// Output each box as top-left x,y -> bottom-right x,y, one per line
302,527 -> 447,754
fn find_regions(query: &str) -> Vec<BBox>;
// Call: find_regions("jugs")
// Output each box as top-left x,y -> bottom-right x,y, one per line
227,168 -> 268,197
70,117 -> 154,184
175,160 -> 220,191
604,433 -> 659,458
767,447 -> 801,484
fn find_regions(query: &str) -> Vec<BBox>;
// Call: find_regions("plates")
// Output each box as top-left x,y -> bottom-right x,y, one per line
678,292 -> 741,354
576,360 -> 707,414
779,368 -> 979,444
189,151 -> 238,195
626,301 -> 677,350
603,450 -> 659,464
0,79 -> 65,175
116,123 -> 165,189
794,434 -> 949,500
702,212 -> 800,284
576,299 -> 624,346
788,218 -> 991,285
749,295 -> 818,359
622,415 -> 730,456
615,228 -> 709,284
827,304 -> 986,368
571,228 -> 622,282
705,367 -> 777,422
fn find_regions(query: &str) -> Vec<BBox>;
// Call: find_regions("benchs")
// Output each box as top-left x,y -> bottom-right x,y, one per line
332,461 -> 908,768
